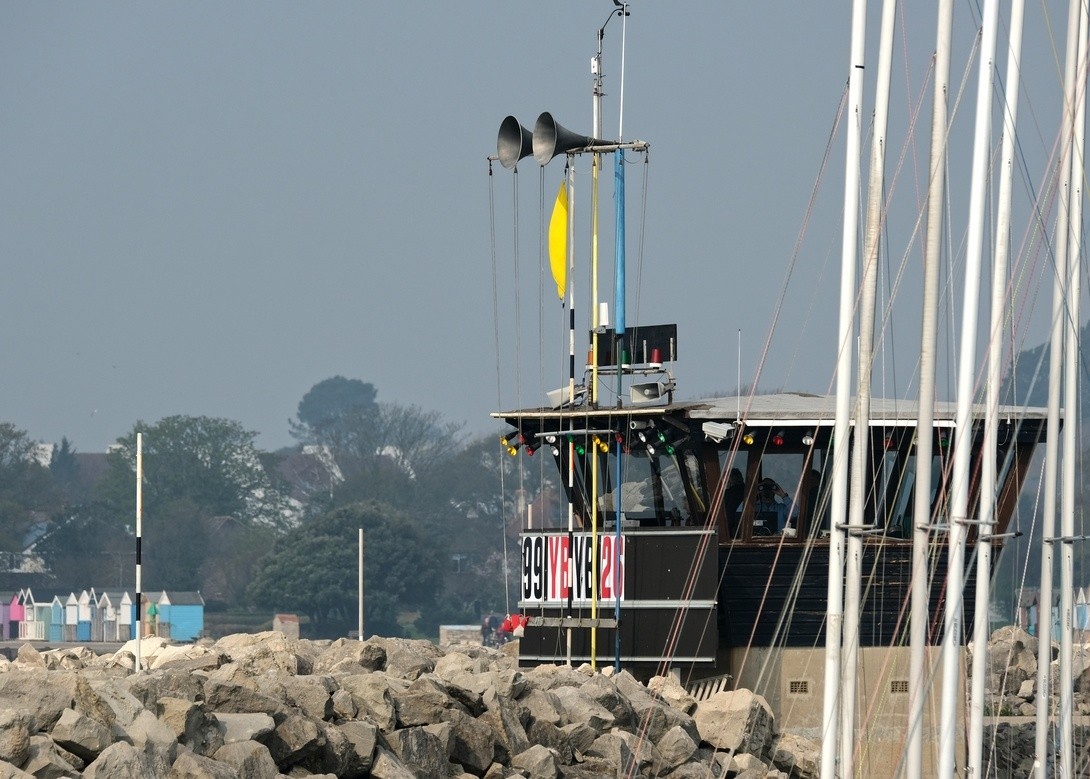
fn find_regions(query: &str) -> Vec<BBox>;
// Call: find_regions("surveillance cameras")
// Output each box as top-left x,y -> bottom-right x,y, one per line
701,420 -> 736,439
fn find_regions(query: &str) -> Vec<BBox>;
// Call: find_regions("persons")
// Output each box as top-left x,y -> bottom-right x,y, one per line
736,478 -> 799,534
723,468 -> 746,512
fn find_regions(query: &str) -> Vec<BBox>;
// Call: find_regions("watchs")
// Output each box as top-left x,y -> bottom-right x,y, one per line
782,492 -> 788,499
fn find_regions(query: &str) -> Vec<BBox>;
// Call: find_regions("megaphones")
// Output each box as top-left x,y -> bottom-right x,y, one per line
532,112 -> 632,165
628,381 -> 664,404
547,385 -> 582,409
496,115 -> 533,169
